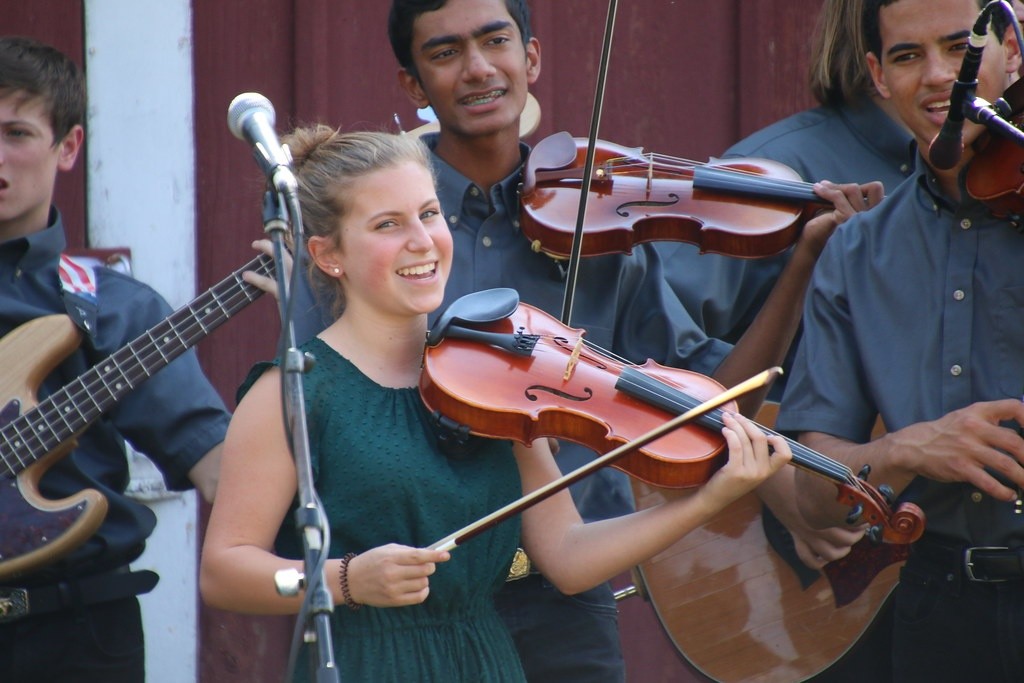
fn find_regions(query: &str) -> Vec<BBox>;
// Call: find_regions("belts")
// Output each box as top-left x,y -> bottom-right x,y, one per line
0,564 -> 160,622
915,541 -> 1024,583
506,548 -> 540,583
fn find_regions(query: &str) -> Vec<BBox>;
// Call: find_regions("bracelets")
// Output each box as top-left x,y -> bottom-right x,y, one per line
340,551 -> 359,606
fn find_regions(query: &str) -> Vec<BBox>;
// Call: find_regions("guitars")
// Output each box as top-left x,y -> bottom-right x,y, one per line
0,221 -> 309,575
623,398 -> 906,683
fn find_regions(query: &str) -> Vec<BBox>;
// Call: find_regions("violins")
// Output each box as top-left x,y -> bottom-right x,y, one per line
963,78 -> 1024,217
417,285 -> 929,553
515,129 -> 884,263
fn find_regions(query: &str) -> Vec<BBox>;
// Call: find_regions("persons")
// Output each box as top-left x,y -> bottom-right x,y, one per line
201,120 -> 795,680
0,37 -> 230,683
287,2 -> 883,683
661,3 -> 923,404
776,2 -> 1022,682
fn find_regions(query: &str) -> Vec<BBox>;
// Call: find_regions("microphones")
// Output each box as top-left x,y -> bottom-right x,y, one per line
929,5 -> 993,170
226,91 -> 299,202
